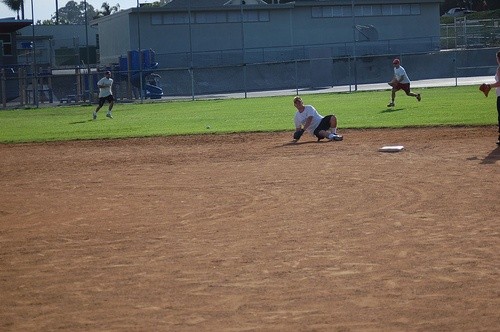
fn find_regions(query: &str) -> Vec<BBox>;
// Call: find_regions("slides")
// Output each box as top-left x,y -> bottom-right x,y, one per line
130,70 -> 164,99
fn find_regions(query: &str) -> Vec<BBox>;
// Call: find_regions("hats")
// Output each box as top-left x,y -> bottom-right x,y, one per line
393,59 -> 400,64
293,97 -> 302,102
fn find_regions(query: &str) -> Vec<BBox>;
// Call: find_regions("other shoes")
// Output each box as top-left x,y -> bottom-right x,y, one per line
416,94 -> 421,101
106,114 -> 113,119
333,135 -> 344,141
386,103 -> 395,107
93,113 -> 96,119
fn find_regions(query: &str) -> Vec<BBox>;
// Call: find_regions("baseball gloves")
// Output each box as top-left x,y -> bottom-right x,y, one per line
479,83 -> 491,97
294,129 -> 304,140
388,79 -> 399,88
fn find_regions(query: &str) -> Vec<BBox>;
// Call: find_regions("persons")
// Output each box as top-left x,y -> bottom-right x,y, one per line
387,59 -> 421,107
93,71 -> 113,119
479,51 -> 500,145
290,97 -> 343,141
